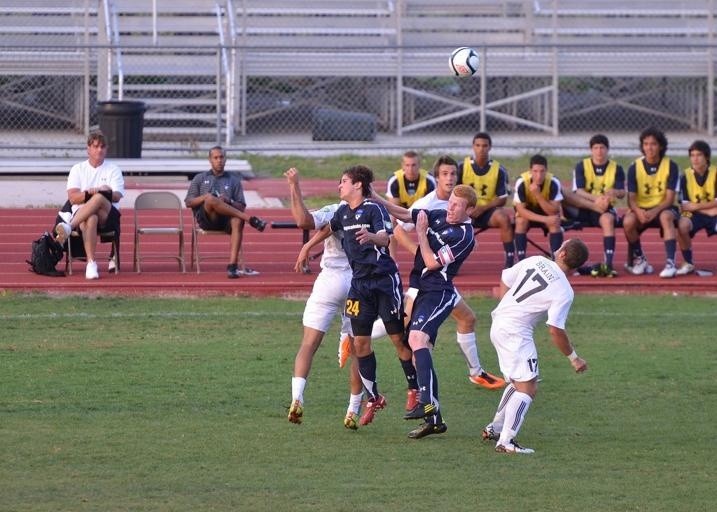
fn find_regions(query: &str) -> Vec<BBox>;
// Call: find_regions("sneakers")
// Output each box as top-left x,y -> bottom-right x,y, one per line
407,419 -> 447,439
624,252 -> 654,275
55,222 -> 71,243
289,401 -> 304,425
85,261 -> 98,279
481,422 -> 535,454
468,368 -> 506,390
226,262 -> 240,278
660,260 -> 677,278
403,386 -> 438,420
337,332 -> 351,369
600,264 -> 615,278
249,215 -> 268,233
676,262 -> 695,275
345,393 -> 387,430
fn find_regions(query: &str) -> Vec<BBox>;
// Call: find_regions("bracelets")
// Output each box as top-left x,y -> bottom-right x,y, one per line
566,351 -> 579,361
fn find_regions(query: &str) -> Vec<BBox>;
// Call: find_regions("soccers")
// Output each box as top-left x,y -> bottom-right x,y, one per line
448,46 -> 480,78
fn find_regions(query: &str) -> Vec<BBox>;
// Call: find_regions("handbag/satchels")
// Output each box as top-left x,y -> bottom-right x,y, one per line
30,232 -> 64,274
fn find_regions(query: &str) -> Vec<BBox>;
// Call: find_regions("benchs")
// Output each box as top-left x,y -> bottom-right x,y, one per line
272,220 -> 706,275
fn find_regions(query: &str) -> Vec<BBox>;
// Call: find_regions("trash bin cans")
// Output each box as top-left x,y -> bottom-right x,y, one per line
96,101 -> 144,159
310,106 -> 374,141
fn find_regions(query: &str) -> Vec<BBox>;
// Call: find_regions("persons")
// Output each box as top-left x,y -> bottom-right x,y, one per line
513,155 -> 564,261
622,128 -> 685,278
562,134 -> 625,276
283,134 -> 514,438
481,239 -> 587,453
55,130 -> 126,279
674,140 -> 717,276
185,146 -> 267,278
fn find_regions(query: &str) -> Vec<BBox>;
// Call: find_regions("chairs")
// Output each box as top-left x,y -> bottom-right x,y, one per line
134,192 -> 185,274
190,209 -> 245,275
67,230 -> 120,276
